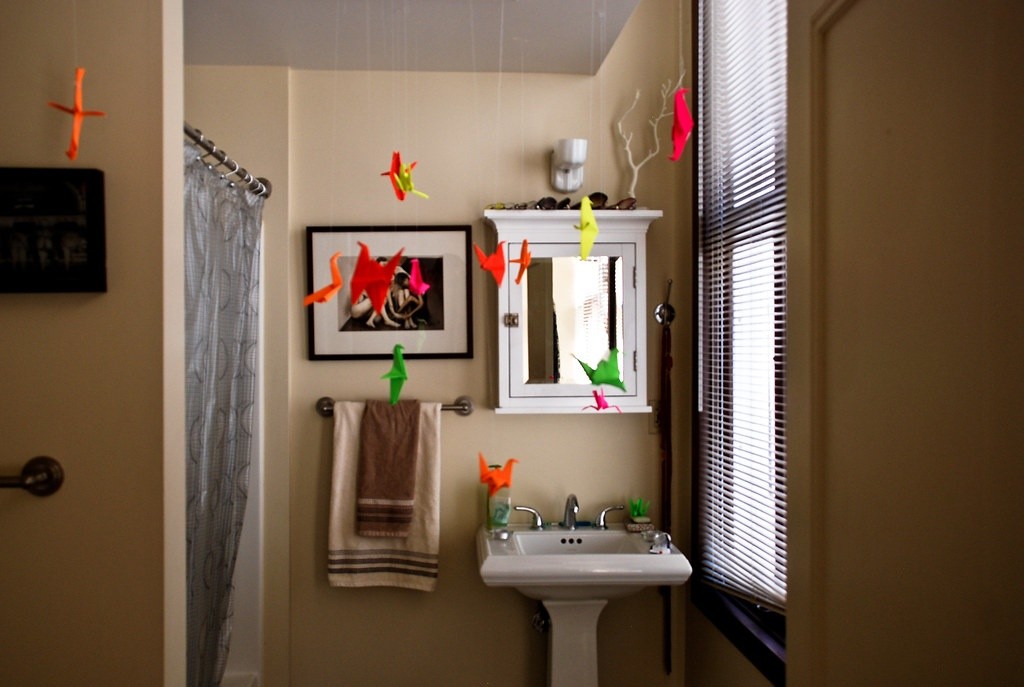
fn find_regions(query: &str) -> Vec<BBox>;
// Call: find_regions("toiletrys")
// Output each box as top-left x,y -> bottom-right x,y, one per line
486,476 -> 514,542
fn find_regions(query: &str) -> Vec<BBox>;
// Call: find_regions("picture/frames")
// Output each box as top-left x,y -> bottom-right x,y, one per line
304,224 -> 474,363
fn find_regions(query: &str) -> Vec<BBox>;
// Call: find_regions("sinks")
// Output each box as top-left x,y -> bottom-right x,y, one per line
473,522 -> 694,604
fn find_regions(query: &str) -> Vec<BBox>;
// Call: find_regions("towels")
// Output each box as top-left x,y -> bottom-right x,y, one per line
326,400 -> 445,595
353,397 -> 423,540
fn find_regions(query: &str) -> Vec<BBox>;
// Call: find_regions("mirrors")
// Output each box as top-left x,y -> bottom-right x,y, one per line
485,205 -> 665,416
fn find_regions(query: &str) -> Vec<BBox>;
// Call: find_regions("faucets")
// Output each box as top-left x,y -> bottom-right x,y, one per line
559,491 -> 580,531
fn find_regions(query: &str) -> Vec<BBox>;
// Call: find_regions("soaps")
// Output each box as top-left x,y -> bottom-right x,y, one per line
633,514 -> 650,524
